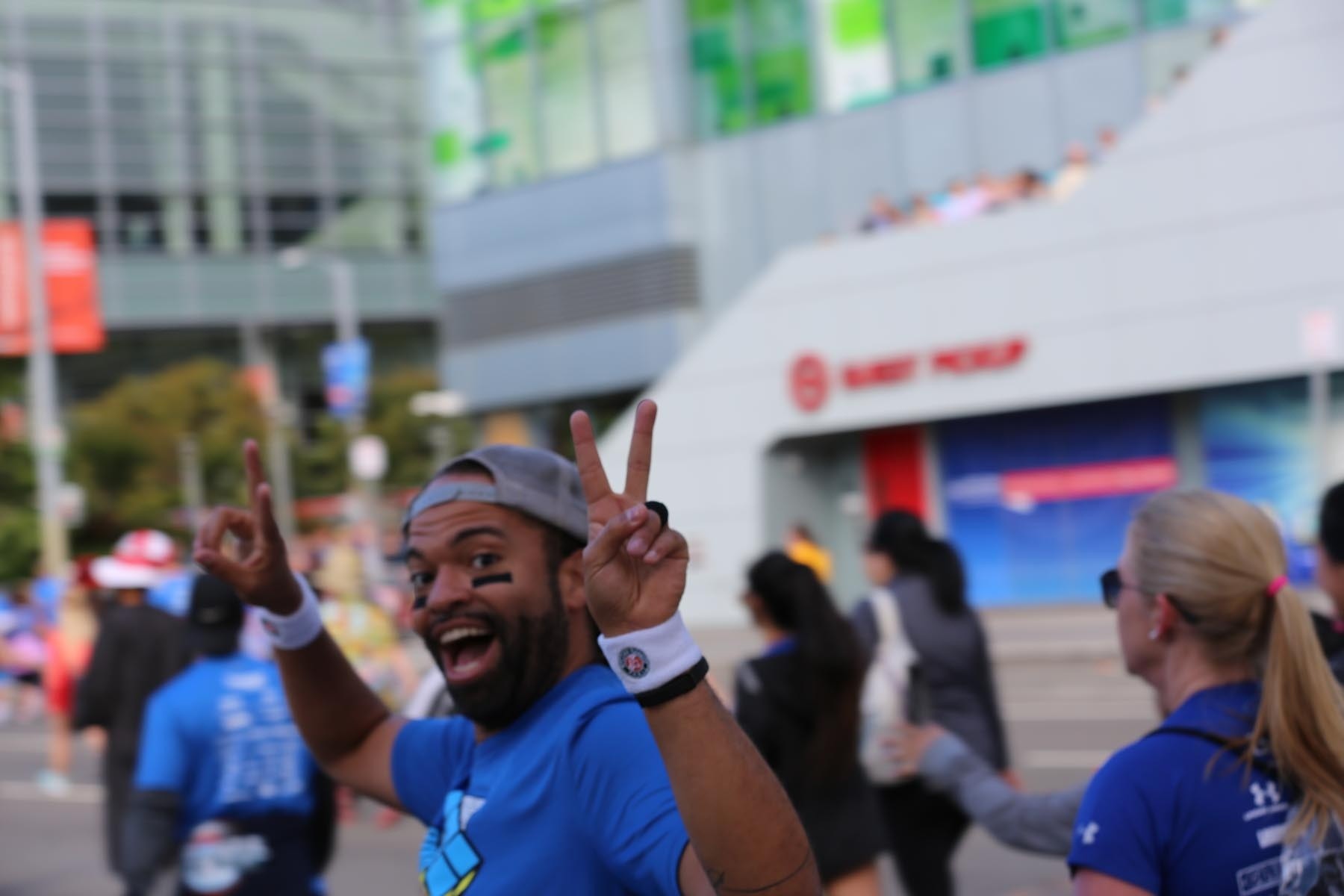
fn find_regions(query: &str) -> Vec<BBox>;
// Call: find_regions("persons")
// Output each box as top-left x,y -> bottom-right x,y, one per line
1,398 -> 1344,896
190,398 -> 823,896
1065,489 -> 1344,896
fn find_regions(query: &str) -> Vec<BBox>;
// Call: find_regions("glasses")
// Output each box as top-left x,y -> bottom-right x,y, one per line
1101,570 -> 1197,626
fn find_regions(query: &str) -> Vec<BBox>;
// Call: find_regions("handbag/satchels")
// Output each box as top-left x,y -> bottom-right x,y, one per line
860,590 -> 923,786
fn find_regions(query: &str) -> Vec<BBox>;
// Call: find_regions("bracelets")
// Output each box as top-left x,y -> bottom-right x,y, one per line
634,656 -> 709,708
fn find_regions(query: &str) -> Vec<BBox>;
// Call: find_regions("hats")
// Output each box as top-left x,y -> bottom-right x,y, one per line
311,545 -> 363,599
89,529 -> 179,588
401,443 -> 589,540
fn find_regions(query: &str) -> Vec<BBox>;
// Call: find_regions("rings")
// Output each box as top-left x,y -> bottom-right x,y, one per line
645,501 -> 668,528
